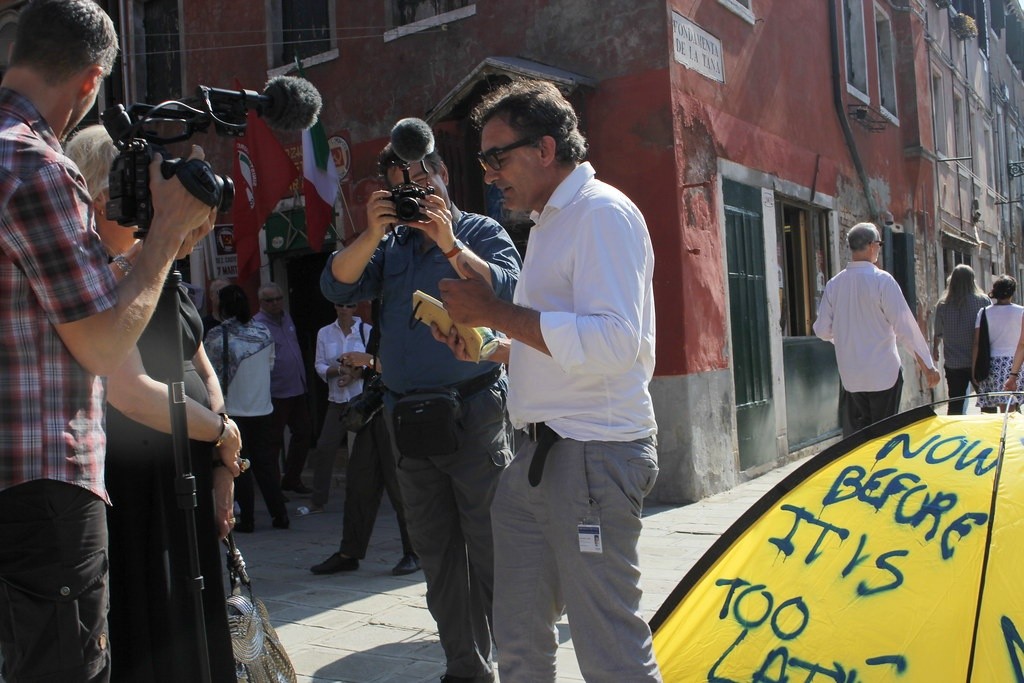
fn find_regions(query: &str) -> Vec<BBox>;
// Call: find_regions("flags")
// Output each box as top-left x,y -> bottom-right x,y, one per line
235,62 -> 338,325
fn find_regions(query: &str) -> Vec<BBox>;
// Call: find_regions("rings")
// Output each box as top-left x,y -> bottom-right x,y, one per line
228,518 -> 235,524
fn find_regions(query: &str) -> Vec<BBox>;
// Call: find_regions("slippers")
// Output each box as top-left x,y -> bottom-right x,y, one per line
295,504 -> 325,515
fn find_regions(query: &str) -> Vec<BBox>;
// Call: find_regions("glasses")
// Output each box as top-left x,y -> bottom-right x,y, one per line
874,240 -> 885,246
338,303 -> 357,308
476,137 -> 533,171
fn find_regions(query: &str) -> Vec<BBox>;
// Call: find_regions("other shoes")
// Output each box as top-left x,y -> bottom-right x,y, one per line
235,521 -> 255,532
273,514 -> 289,529
282,480 -> 313,498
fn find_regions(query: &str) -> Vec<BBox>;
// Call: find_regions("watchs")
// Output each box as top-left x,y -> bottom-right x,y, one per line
370,359 -> 375,365
442,239 -> 464,258
1010,373 -> 1017,376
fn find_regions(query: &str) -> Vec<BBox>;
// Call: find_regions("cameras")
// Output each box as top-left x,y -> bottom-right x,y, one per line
385,185 -> 432,221
101,102 -> 235,237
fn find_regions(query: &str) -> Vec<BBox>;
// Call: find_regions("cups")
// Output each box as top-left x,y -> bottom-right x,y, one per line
477,325 -> 500,358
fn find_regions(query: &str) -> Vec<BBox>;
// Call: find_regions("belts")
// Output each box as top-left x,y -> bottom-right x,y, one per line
528,421 -> 562,486
382,363 -> 504,401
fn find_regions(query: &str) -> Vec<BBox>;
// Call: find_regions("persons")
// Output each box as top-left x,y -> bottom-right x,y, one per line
0,0 -> 218,683
594,535 -> 599,546
65,125 -> 241,683
972,274 -> 1024,413
200,279 -> 422,576
933,264 -> 993,415
320,143 -> 523,683
813,223 -> 940,441
438,80 -> 663,683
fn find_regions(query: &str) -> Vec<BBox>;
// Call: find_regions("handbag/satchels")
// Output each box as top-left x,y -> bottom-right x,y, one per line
223,533 -> 299,683
342,373 -> 391,431
975,311 -> 991,380
395,388 -> 464,460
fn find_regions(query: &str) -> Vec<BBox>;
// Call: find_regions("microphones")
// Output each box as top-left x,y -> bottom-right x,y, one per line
390,118 -> 436,162
197,75 -> 322,132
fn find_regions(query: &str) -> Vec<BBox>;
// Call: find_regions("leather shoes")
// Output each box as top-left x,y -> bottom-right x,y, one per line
392,551 -> 421,574
310,554 -> 360,574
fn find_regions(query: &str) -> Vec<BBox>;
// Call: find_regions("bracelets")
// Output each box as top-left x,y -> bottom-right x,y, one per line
112,255 -> 133,280
338,366 -> 343,376
215,413 -> 229,448
